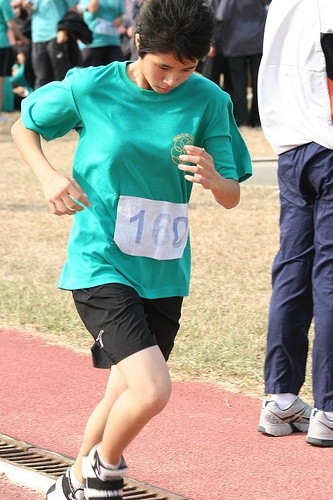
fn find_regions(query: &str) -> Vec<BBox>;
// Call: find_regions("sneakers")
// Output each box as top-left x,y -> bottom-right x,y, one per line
46,443 -> 128,500
257,398 -> 333,447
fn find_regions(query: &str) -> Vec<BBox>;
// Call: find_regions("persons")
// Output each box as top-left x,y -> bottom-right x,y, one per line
257,0 -> 333,448
83,0 -> 127,70
10,0 -> 36,45
10,48 -> 34,108
127,0 -> 148,63
30,0 -> 81,91
217,0 -> 270,127
197,0 -> 220,88
0,0 -> 28,110
9,0 -> 252,500
